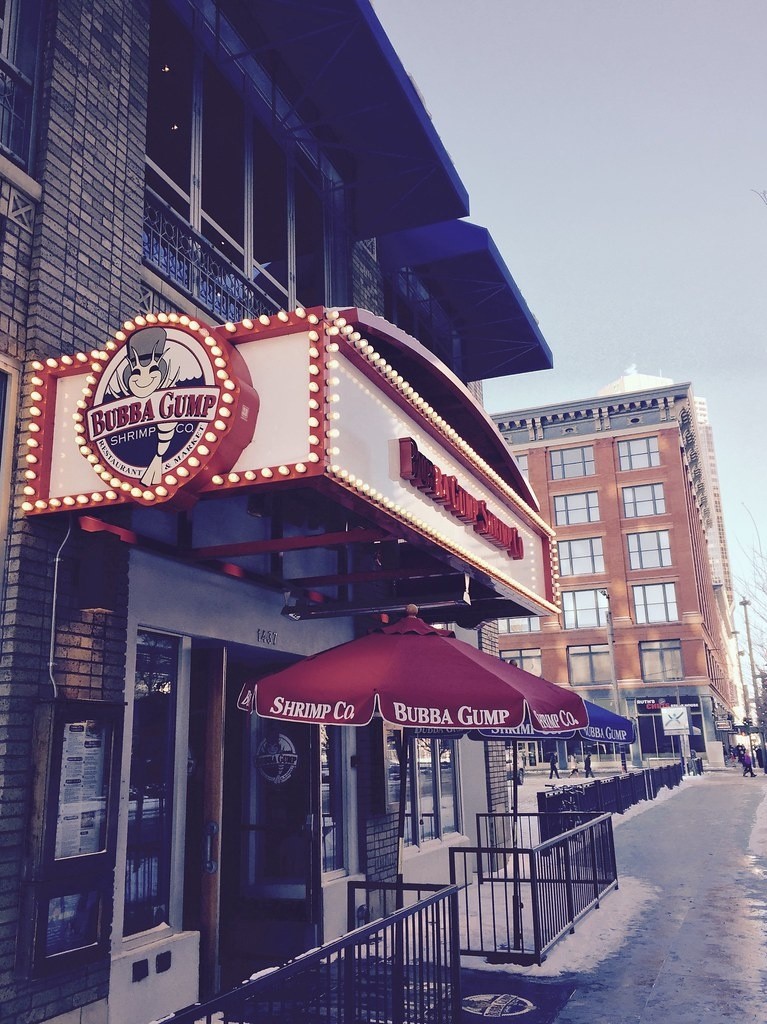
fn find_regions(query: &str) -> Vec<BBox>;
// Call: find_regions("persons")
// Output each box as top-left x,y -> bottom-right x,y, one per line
568,751 -> 581,779
722,742 -> 767,769
548,751 -> 562,779
584,754 -> 596,778
742,749 -> 758,778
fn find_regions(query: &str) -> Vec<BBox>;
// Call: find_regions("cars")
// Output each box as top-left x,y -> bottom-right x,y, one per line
390,755 -> 451,783
505,748 -> 524,786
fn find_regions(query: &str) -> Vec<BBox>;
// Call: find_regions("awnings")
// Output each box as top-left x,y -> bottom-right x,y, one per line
164,0 -> 470,241
376,220 -> 554,387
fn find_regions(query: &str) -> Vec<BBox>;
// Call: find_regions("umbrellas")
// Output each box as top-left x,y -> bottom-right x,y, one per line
236,603 -> 639,910
